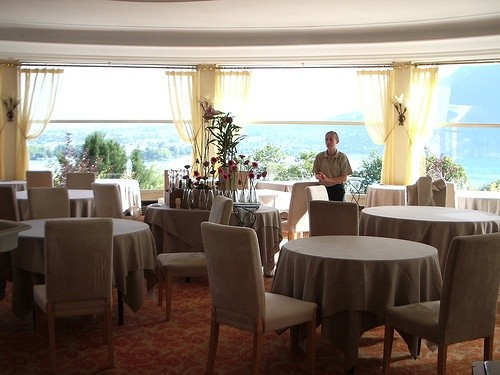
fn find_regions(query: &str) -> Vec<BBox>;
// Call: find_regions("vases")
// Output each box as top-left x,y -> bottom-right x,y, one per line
206,190 -> 214,209
218,190 -> 223,197
198,190 -> 206,209
253,189 -> 257,203
225,189 -> 231,198
231,191 -> 238,203
183,189 -> 191,210
248,190 -> 253,204
240,190 -> 246,203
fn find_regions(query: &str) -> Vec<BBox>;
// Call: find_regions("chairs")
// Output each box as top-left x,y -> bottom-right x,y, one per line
0,168 -> 500,375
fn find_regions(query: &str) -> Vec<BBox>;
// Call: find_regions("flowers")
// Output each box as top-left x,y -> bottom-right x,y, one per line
171,97 -> 268,191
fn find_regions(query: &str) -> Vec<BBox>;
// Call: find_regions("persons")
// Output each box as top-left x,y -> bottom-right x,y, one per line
312,132 -> 353,201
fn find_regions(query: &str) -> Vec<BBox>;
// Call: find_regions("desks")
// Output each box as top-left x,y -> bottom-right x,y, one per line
362,205 -> 500,279
16,188 -> 96,221
365,184 -> 407,208
256,180 -> 294,193
273,234 -> 442,374
144,200 -> 283,276
14,216 -> 160,325
455,191 -> 500,214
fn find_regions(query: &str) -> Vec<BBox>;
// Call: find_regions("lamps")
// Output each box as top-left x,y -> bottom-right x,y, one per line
3,96 -> 20,122
384,93 -> 412,144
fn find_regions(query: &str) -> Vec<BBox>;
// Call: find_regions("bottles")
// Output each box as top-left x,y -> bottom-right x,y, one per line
174,198 -> 182,209
